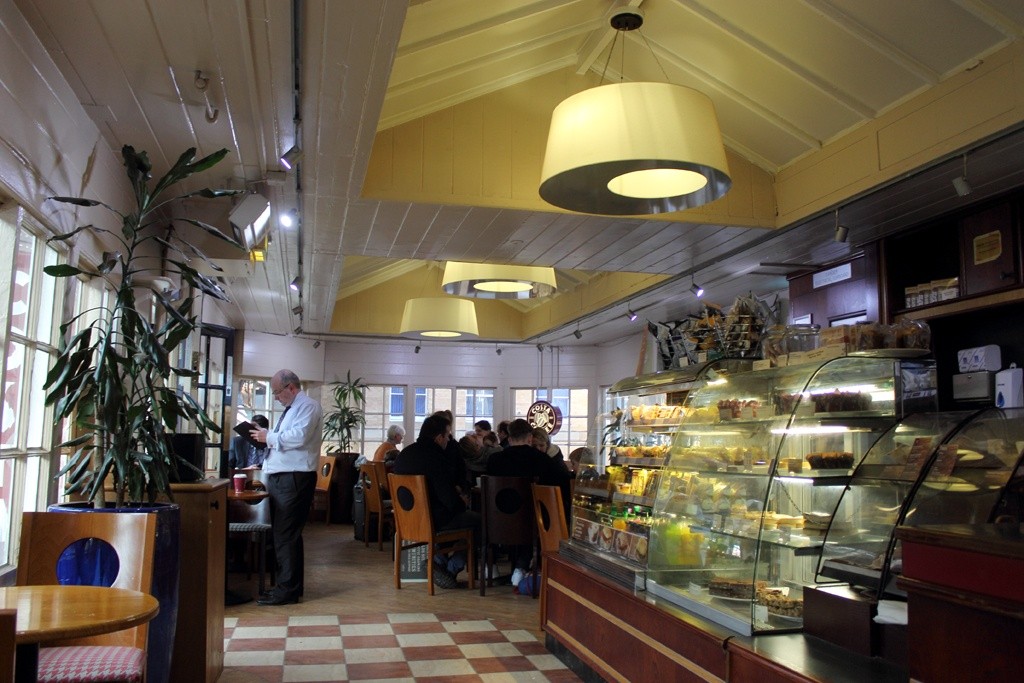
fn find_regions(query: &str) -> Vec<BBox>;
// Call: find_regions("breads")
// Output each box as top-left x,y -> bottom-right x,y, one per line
706,577 -> 804,617
743,510 -> 851,538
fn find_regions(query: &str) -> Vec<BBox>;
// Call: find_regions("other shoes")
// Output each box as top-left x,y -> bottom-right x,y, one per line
255,594 -> 295,606
487,576 -> 504,585
424,560 -> 459,589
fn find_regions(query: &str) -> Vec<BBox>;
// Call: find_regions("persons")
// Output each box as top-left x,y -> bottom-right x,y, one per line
227,369 -> 322,605
376,409 -> 598,588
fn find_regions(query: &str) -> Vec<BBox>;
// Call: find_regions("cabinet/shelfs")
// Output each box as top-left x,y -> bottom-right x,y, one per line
576,414 -> 1002,544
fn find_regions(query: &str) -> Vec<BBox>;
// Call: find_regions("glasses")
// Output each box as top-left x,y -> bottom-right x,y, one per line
273,384 -> 290,397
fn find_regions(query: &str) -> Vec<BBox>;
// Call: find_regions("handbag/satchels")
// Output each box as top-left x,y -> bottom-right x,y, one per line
519,572 -> 542,599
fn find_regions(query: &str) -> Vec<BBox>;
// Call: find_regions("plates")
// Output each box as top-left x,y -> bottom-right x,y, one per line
754,604 -> 804,622
709,593 -> 758,602
813,469 -> 852,476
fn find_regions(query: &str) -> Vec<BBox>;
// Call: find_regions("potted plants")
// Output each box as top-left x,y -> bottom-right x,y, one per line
38,147 -> 248,683
321,369 -> 369,479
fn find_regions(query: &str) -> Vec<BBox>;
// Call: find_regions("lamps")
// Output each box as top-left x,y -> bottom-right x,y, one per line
281,208 -> 301,227
539,12 -> 733,216
689,273 -> 704,297
290,277 -> 303,290
573,321 -> 582,339
280,118 -> 305,170
835,210 -> 848,243
440,261 -> 558,298
626,300 -> 637,322
399,298 -> 479,341
292,305 -> 320,349
951,155 -> 973,197
414,339 -> 544,355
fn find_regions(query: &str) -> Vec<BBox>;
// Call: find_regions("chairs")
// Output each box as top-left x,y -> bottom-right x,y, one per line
16,512 -> 157,683
315,456 -> 337,526
388,473 -> 475,596
231,470 -> 276,596
478,474 -> 568,596
360,461 -> 392,551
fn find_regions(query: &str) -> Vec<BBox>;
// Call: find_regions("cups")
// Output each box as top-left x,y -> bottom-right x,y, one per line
233,474 -> 247,493
787,458 -> 802,474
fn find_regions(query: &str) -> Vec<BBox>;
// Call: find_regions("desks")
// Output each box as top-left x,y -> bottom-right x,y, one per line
0,586 -> 160,683
227,487 -> 269,499
65,477 -> 230,683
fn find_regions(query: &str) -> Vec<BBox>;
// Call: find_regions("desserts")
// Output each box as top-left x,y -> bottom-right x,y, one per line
718,394 -> 803,421
805,452 -> 855,469
812,391 -> 872,412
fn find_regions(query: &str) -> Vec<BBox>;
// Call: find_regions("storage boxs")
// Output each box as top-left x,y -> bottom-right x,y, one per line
952,371 -> 992,399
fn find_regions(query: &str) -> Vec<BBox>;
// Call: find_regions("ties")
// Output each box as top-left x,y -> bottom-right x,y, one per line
253,447 -> 259,464
263,405 -> 291,459
